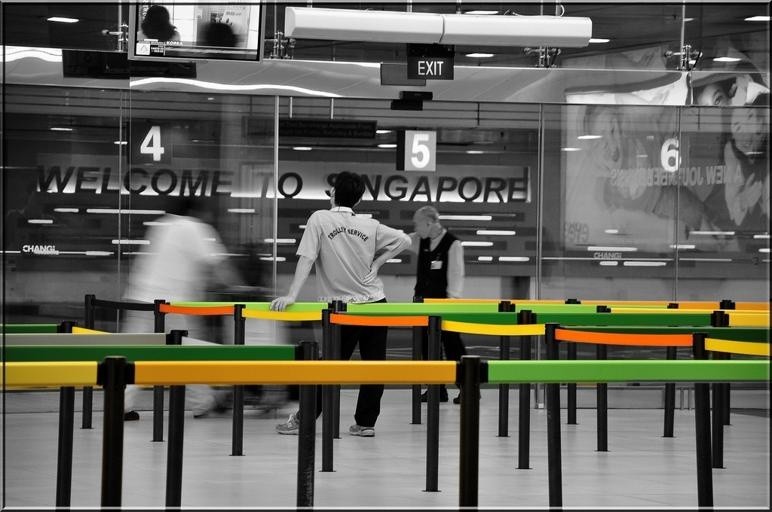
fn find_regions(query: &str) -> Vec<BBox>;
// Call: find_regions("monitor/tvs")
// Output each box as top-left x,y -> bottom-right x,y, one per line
126,3 -> 267,65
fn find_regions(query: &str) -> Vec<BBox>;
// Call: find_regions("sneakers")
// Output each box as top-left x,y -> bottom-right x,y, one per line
421,389 -> 448,402
349,425 -> 374,437
275,414 -> 300,435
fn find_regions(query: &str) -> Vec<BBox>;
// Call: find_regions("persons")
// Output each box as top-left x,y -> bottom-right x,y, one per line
121,180 -> 248,422
270,171 -> 411,436
404,205 -> 468,404
137,5 -> 180,43
704,107 -> 770,261
692,83 -> 741,108
580,102 -> 726,255
